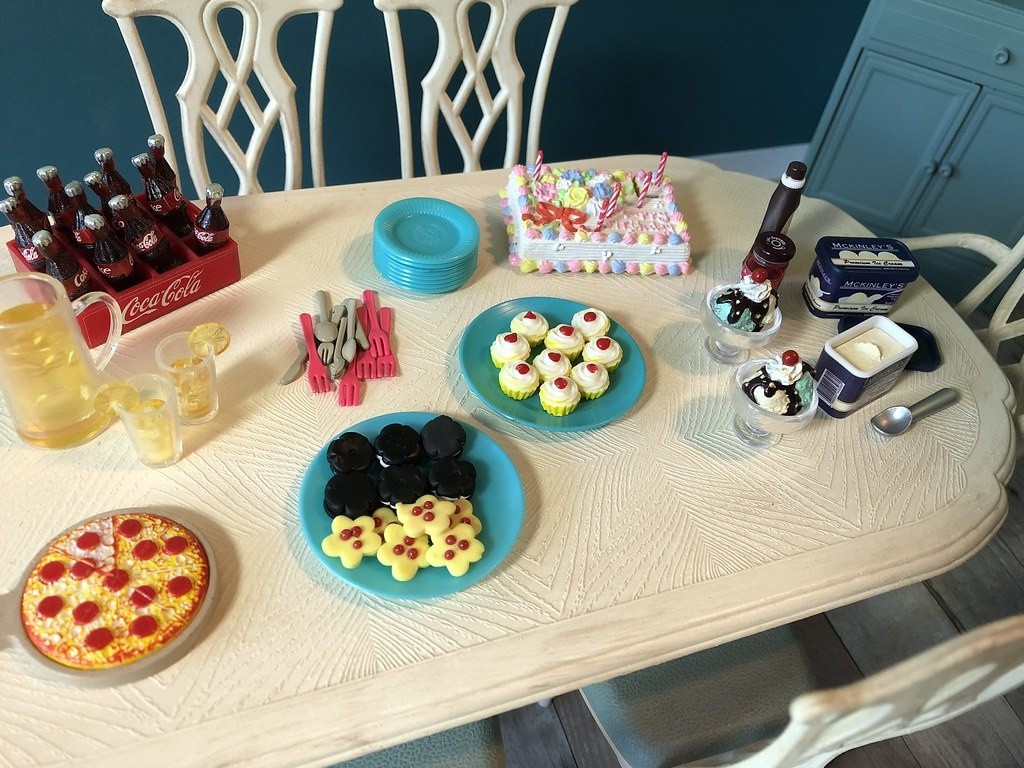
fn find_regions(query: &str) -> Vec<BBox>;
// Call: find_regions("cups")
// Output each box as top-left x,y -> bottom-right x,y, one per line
155,331 -> 220,425
109,374 -> 183,468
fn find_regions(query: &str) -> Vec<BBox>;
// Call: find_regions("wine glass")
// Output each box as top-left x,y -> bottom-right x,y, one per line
729,359 -> 820,449
699,285 -> 782,366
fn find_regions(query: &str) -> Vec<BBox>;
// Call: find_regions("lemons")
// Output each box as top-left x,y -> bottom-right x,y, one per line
188,323 -> 230,358
93,381 -> 139,417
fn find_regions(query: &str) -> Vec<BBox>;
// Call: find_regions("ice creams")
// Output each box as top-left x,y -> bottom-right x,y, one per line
832,328 -> 907,373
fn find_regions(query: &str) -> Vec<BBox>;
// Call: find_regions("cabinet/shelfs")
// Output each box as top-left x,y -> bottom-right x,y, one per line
800,0 -> 1024,318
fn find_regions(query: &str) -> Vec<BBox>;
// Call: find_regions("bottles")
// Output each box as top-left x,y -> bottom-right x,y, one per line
757,161 -> 807,246
0,133 -> 192,301
194,182 -> 230,257
742,231 -> 796,290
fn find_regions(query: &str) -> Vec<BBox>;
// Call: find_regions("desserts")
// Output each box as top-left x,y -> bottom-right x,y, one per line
711,268 -> 780,334
742,350 -> 816,416
320,415 -> 485,582
489,307 -> 624,418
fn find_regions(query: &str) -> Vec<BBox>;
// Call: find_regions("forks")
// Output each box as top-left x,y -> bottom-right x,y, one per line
300,313 -> 331,393
317,305 -> 344,365
339,289 -> 395,406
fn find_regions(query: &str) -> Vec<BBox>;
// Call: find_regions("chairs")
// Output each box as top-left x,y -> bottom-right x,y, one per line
577,613 -> 1024,767
883,234 -> 1023,458
102,0 -> 345,202
371,0 -> 581,182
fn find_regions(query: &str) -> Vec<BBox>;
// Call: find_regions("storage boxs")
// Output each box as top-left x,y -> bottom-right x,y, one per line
803,235 -> 920,318
7,188 -> 241,348
812,313 -> 918,421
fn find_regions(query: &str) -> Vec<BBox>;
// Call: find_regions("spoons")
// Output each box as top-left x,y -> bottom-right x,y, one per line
870,388 -> 958,436
342,300 -> 357,363
315,290 -> 338,341
334,317 -> 347,378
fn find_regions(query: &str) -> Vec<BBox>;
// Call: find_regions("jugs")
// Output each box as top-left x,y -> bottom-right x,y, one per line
0,272 -> 123,449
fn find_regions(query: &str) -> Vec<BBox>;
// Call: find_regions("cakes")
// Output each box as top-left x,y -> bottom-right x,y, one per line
499,164 -> 692,278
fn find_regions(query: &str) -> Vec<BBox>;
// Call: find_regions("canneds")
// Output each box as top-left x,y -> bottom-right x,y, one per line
737,230 -> 796,296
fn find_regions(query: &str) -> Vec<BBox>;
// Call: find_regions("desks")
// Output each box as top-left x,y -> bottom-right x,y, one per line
0,151 -> 1015,768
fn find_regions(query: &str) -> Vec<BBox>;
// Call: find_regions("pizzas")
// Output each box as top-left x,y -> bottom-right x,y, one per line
21,513 -> 209,670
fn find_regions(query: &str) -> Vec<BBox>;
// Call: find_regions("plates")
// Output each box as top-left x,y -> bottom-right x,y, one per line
375,197 -> 480,295
458,296 -> 646,431
300,412 -> 525,599
